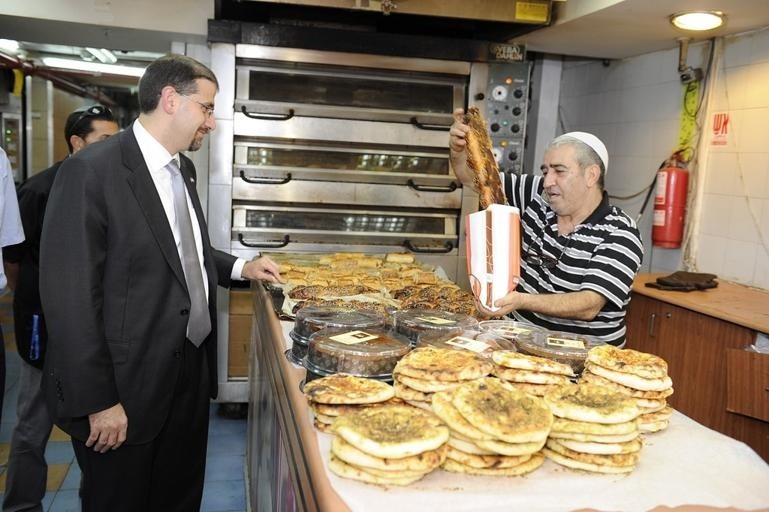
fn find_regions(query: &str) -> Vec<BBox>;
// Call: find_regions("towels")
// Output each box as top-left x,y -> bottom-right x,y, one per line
645,273 -> 718,291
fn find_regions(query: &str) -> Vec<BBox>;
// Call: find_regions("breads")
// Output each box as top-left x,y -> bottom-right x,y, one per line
456,106 -> 508,208
259,254 -> 609,384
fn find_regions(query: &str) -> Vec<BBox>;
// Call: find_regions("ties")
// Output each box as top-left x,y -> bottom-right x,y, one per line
165,160 -> 212,347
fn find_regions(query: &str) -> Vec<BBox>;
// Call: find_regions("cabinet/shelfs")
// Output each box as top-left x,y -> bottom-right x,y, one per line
626,270 -> 769,465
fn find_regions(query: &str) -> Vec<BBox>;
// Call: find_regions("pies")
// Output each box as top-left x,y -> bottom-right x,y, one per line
302,343 -> 675,485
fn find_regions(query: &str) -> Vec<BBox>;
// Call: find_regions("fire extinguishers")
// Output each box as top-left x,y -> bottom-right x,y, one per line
635,146 -> 695,248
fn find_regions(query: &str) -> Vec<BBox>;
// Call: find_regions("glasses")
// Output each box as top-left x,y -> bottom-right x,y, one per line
70,106 -> 114,134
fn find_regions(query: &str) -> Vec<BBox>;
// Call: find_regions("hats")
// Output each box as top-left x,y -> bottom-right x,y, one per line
565,131 -> 608,175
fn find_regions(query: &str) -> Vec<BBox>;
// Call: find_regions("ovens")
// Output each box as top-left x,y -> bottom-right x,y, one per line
213,38 -> 533,402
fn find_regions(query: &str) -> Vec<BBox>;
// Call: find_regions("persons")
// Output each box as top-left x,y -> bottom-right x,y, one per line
0,143 -> 27,460
0,105 -> 119,512
38,54 -> 293,512
448,108 -> 644,350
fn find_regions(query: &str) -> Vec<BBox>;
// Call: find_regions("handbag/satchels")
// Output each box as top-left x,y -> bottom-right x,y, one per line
646,271 -> 718,290
